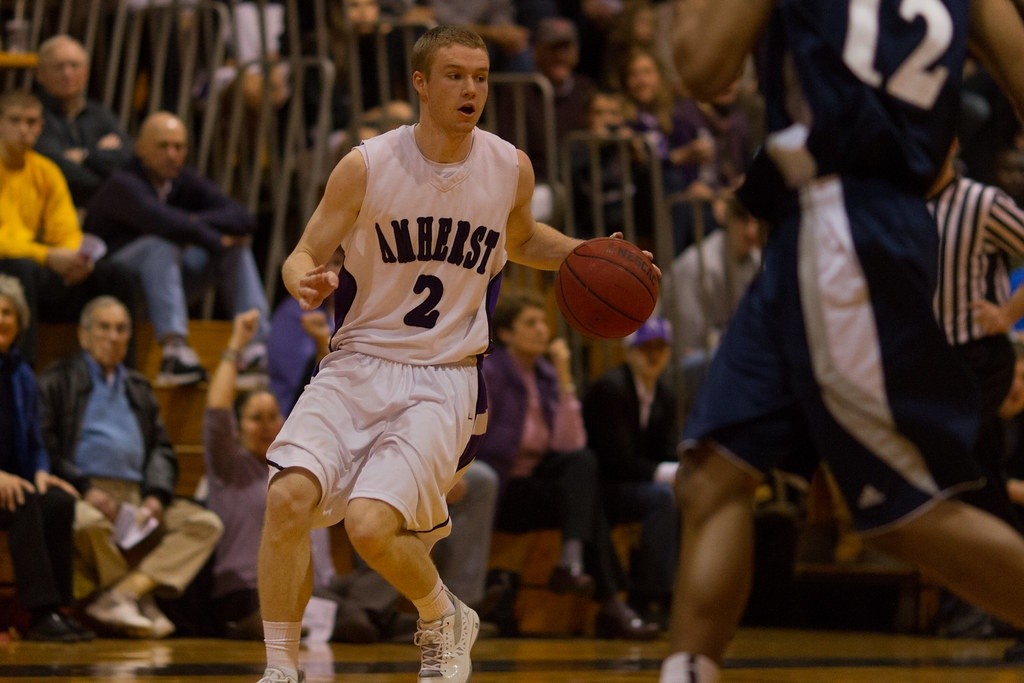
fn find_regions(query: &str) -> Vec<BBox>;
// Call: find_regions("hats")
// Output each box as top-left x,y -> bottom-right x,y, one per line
624,318 -> 674,349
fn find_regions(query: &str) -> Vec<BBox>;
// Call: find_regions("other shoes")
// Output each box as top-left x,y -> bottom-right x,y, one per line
28,611 -> 96,642
595,605 -> 659,639
156,353 -> 207,389
547,572 -> 593,593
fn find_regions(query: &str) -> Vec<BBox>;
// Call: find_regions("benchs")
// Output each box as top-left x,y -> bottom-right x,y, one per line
30,313 -> 937,635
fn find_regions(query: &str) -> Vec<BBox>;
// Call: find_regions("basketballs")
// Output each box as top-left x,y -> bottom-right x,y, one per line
554,236 -> 659,342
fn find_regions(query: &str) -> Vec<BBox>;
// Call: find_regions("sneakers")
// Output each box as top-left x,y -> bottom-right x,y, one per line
257,668 -> 299,683
412,587 -> 481,683
125,595 -> 176,640
83,591 -> 152,637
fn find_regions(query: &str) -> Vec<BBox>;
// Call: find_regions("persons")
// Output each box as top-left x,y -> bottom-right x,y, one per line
256,23 -> 662,683
927,137 -> 1024,659
654,0 -> 1024,683
0,0 -> 1024,642
